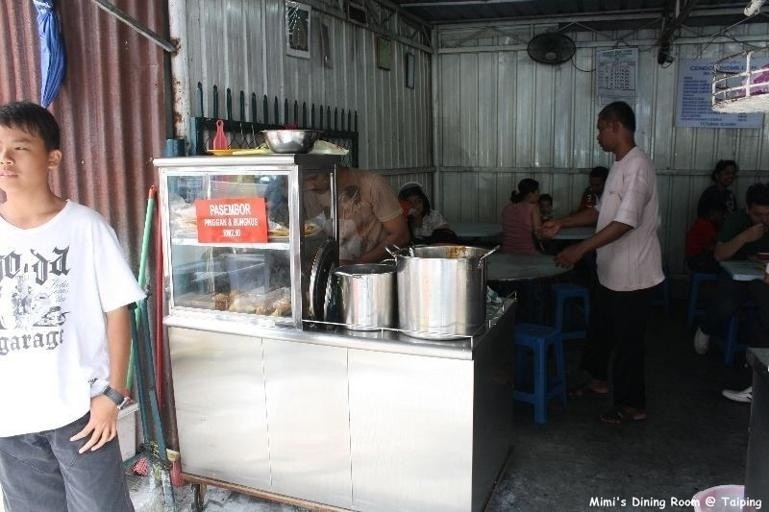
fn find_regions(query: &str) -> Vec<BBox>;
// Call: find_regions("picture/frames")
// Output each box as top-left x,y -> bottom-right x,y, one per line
284,0 -> 311,60
376,36 -> 393,70
405,52 -> 416,90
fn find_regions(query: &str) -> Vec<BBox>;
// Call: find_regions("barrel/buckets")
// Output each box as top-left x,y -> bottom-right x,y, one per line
691,484 -> 745,512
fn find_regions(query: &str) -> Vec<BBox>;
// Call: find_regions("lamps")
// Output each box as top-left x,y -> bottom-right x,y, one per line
658,7 -> 673,69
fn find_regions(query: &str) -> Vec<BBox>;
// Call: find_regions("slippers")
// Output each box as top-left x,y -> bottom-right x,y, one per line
601,408 -> 647,425
573,388 -> 610,403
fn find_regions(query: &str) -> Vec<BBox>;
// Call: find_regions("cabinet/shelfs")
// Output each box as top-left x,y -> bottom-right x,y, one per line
151,152 -> 342,336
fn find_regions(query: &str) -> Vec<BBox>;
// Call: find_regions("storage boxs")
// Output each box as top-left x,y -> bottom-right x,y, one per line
115,403 -> 141,464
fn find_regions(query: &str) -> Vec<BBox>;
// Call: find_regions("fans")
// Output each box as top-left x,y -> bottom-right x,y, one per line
527,31 -> 576,65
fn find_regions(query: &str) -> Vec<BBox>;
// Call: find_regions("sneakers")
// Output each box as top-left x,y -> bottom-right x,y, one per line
722,386 -> 753,403
694,328 -> 710,355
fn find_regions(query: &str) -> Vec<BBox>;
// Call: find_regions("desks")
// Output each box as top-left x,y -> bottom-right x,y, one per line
744,345 -> 769,512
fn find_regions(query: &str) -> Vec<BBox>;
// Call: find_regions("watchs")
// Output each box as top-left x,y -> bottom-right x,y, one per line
105,387 -> 132,411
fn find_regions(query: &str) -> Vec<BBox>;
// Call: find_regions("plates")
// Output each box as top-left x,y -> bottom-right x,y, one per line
207,150 -> 251,155
269,229 -> 317,235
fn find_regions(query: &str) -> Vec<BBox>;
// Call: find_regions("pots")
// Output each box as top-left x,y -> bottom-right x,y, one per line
335,257 -> 397,332
394,245 -> 488,341
343,329 -> 471,351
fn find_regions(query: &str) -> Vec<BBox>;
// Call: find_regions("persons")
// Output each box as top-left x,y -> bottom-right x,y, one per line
501,178 -> 544,255
720,183 -> 769,404
299,157 -> 411,267
685,186 -> 723,276
0,101 -> 147,511
539,193 -> 553,222
696,160 -> 738,233
568,165 -> 609,216
397,180 -> 457,245
534,101 -> 666,425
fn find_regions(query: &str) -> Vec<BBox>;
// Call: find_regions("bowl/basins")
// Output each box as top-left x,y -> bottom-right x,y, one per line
260,127 -> 322,154
756,250 -> 769,260
